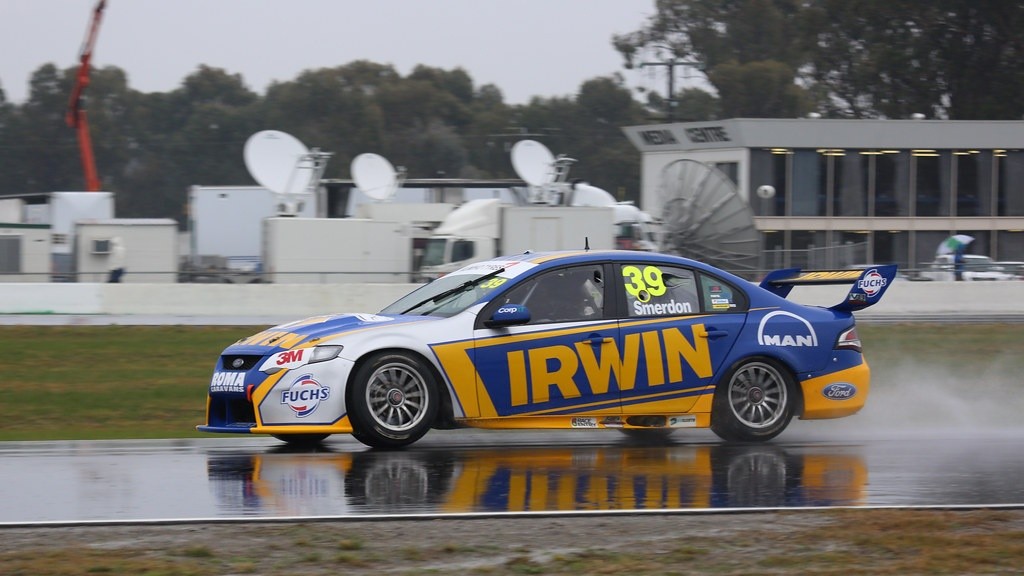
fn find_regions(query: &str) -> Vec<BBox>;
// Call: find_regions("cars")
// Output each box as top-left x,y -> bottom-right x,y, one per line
919,255 -> 1009,280
195,249 -> 900,443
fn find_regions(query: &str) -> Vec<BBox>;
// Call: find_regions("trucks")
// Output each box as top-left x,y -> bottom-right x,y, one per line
412,196 -> 653,283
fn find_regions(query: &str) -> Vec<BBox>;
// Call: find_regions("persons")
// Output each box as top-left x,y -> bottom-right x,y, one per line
526,273 -> 585,322
106,235 -> 127,281
955,250 -> 969,281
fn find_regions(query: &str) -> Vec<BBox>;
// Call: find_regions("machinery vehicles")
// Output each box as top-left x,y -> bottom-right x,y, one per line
65,1 -> 110,192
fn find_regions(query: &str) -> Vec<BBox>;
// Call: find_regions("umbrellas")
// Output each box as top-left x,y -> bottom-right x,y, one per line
936,235 -> 974,258
